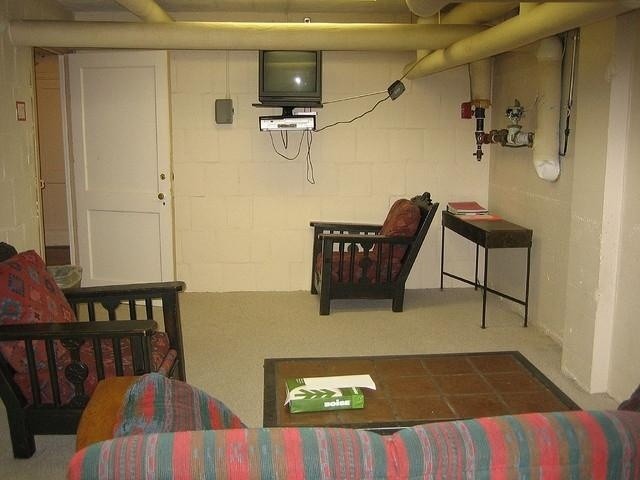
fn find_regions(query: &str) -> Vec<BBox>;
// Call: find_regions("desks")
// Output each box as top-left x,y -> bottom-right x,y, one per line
441,207 -> 533,331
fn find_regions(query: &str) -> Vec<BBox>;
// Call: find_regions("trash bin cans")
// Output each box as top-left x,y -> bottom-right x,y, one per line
46,264 -> 82,321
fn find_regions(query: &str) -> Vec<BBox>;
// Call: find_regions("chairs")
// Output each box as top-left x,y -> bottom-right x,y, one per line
308,189 -> 440,316
1,244 -> 189,459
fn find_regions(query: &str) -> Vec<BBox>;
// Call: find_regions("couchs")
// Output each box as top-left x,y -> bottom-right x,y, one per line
70,376 -> 640,479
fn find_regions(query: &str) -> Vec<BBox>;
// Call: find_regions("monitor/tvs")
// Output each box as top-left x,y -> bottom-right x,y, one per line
258,50 -> 322,103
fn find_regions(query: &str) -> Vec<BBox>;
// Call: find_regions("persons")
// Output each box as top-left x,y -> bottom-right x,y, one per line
447,201 -> 489,215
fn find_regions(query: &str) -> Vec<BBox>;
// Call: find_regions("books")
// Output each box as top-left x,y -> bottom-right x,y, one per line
284,375 -> 366,414
